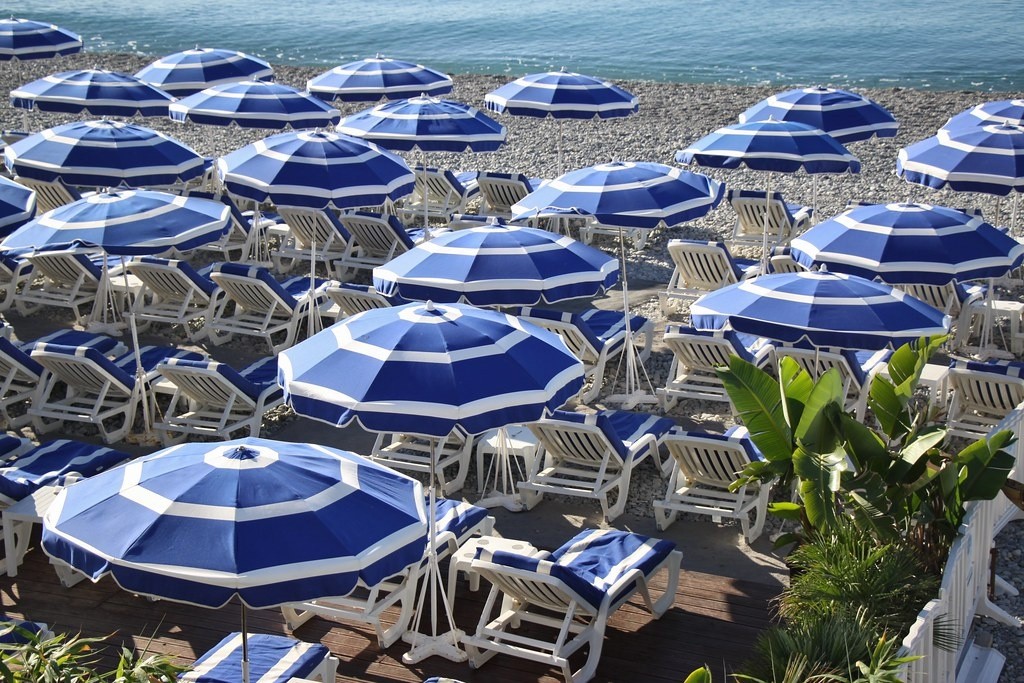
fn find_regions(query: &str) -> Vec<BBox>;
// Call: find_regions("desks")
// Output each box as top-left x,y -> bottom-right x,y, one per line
110,274 -> 158,313
880,360 -> 949,421
321,298 -> 349,318
150,375 -> 200,436
447,535 -> 539,630
263,223 -> 290,262
974,300 -> 1024,353
476,424 -> 539,494
4,485 -> 60,577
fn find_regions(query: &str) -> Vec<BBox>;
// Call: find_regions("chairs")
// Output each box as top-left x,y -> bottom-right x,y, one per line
0,164 -> 1024,683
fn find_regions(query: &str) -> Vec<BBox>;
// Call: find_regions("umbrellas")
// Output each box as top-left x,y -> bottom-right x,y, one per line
337,93 -> 507,241
690,264 -> 953,384
0,15 -> 84,131
4,115 -> 214,324
10,64 -> 179,116
897,120 -> 1024,229
41,437 -> 429,683
0,186 -> 232,434
134,43 -> 276,191
168,75 -> 341,261
484,65 -> 639,176
739,85 -> 901,218
790,199 -> 1024,294
218,127 -> 415,337
674,115 -> 862,274
372,218 -> 620,493
940,99 -> 1023,239
306,53 -> 454,104
277,300 -> 585,638
509,154 -> 727,396
